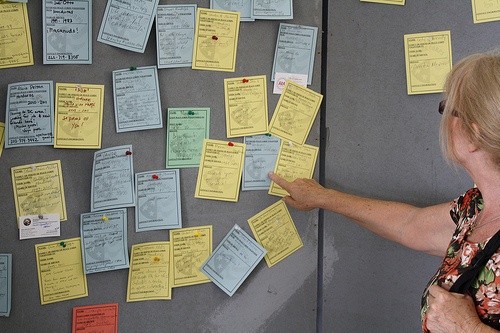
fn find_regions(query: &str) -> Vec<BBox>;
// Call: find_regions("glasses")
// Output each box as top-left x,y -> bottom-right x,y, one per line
439,98 -> 460,118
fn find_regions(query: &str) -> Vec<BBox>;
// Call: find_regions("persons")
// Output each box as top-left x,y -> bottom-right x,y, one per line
267,48 -> 500,333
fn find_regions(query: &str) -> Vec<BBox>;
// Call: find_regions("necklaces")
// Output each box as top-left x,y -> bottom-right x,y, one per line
466,215 -> 500,236
